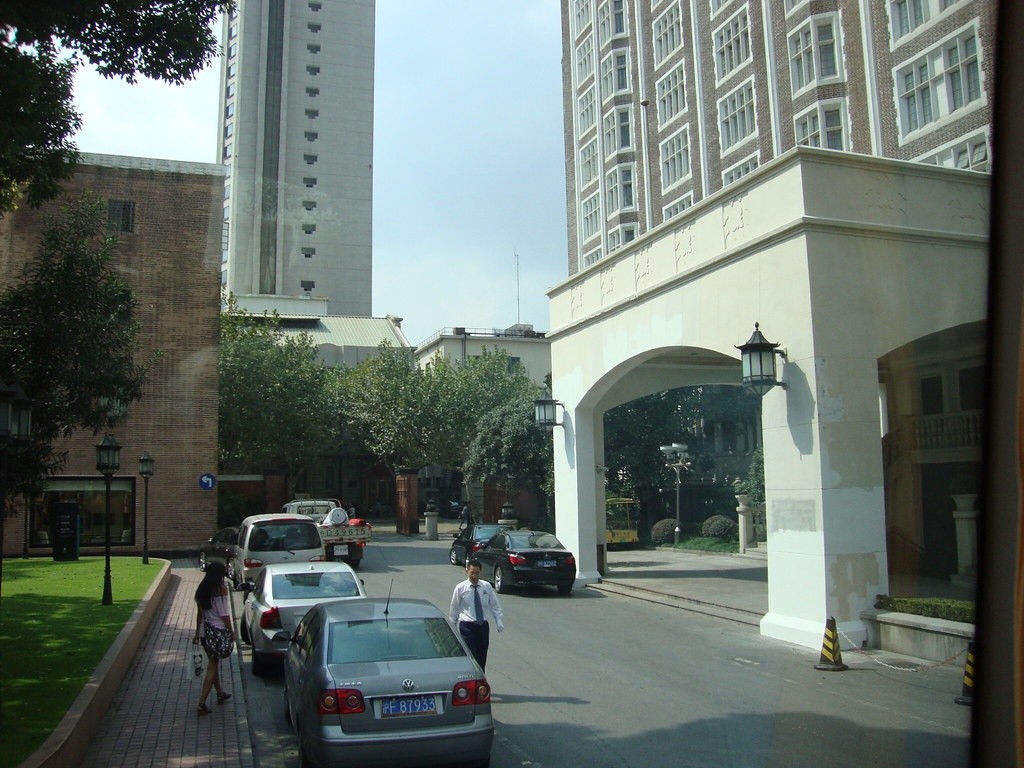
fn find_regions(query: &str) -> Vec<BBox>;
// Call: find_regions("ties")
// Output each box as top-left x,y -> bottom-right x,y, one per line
471,585 -> 484,626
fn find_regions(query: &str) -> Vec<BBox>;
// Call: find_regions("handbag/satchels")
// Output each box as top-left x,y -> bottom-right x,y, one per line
186,643 -> 206,680
204,621 -> 234,659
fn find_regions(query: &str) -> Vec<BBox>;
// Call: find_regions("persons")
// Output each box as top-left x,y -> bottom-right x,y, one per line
459,501 -> 468,531
448,561 -> 503,673
192,562 -> 237,715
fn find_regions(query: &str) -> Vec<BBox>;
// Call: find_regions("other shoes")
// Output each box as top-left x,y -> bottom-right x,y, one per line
197,702 -> 213,716
217,691 -> 232,704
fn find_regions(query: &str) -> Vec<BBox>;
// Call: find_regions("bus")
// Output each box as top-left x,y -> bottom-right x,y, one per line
606,497 -> 640,548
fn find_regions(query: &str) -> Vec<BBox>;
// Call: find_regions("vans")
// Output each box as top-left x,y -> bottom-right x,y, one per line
231,513 -> 325,592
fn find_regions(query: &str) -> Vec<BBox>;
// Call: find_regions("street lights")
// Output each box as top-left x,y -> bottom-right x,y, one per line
94,433 -> 123,605
137,451 -> 157,565
659,443 -> 692,543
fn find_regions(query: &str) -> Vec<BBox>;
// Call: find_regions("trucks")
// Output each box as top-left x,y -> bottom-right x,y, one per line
280,499 -> 371,567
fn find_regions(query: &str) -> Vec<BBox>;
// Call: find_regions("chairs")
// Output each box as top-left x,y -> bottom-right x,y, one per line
250,528 -> 272,552
283,527 -> 305,550
349,639 -> 373,662
402,635 -> 439,656
541,543 -> 552,548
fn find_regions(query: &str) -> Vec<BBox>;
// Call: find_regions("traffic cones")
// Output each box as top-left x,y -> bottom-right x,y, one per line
954,640 -> 973,705
813,616 -> 851,670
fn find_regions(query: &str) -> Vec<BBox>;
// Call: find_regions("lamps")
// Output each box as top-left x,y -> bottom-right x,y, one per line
529,384 -> 565,439
734,321 -> 789,399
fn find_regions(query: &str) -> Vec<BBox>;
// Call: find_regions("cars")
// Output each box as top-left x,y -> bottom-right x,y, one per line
440,499 -> 461,519
449,522 -> 513,567
269,597 -> 492,768
237,561 -> 367,677
197,527 -> 283,580
468,530 -> 575,594
